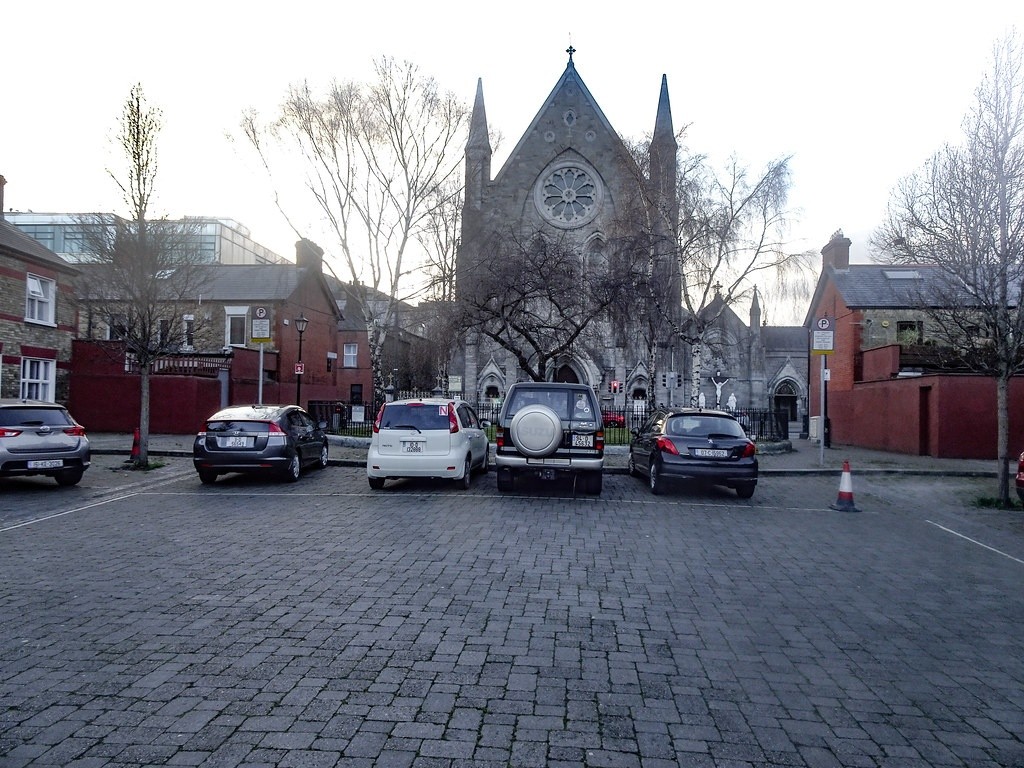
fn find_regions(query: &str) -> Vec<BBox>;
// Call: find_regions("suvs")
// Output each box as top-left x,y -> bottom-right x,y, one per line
495,378 -> 607,496
366,398 -> 492,491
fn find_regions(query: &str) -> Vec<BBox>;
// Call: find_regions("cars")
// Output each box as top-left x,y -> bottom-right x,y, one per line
601,409 -> 625,428
0,396 -> 93,486
627,406 -> 759,499
726,409 -> 753,432
191,403 -> 331,485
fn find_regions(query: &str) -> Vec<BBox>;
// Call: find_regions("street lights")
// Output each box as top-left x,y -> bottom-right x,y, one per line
291,311 -> 311,406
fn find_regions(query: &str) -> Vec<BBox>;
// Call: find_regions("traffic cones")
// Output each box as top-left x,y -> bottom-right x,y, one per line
123,427 -> 148,464
826,460 -> 865,513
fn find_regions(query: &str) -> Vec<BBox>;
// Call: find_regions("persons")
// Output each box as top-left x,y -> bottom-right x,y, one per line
743,413 -> 753,431
711,377 -> 729,403
699,393 -> 706,408
727,393 -> 737,411
